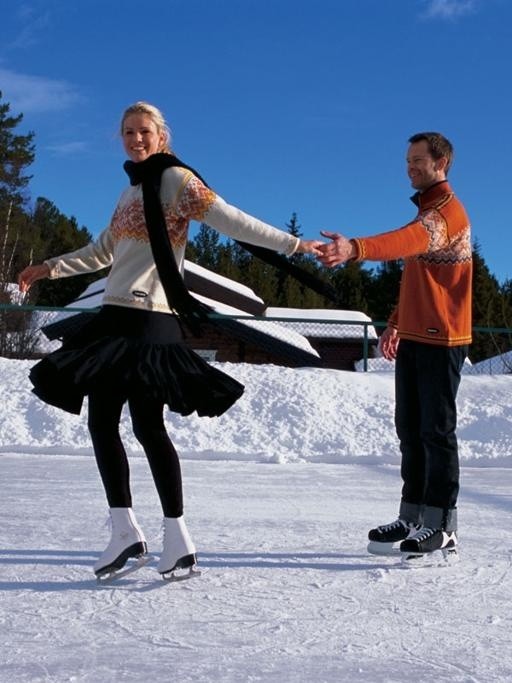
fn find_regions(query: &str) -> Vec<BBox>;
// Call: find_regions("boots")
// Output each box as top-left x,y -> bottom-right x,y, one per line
156,514 -> 198,574
93,506 -> 148,575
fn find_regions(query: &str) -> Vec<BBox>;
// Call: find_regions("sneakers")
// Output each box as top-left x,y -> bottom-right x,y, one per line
399,525 -> 461,554
367,519 -> 424,544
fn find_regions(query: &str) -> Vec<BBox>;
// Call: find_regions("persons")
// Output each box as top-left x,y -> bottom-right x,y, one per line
17,99 -> 331,577
312,129 -> 474,553
